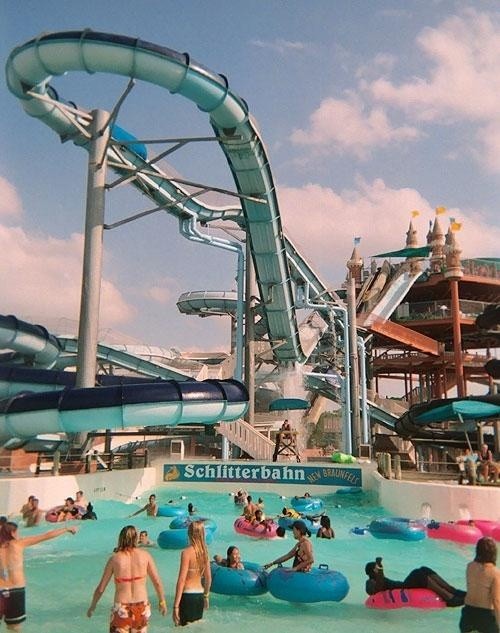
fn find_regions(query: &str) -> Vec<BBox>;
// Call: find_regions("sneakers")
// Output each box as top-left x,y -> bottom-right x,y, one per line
446,590 -> 466,607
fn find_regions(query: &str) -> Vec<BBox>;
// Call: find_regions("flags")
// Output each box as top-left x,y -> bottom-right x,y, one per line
450,221 -> 462,231
437,208 -> 445,216
411,210 -> 419,218
353,236 -> 362,247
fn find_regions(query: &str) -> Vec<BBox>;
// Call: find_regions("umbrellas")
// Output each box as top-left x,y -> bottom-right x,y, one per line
416,401 -> 500,450
267,397 -> 310,422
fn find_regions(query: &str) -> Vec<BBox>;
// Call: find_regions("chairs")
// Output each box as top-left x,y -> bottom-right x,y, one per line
457,455 -> 499,486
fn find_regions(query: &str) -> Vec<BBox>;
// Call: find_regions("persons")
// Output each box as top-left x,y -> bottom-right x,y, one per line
364,556 -> 466,608
458,535 -> 500,632
275,493 -> 310,520
0,521 -> 79,633
280,418 -> 295,443
209,546 -> 245,568
23,489 -> 97,527
234,490 -> 264,525
133,493 -> 158,518
316,514 -> 334,539
458,439 -> 500,488
173,521 -> 212,625
262,520 -> 315,572
87,523 -> 167,633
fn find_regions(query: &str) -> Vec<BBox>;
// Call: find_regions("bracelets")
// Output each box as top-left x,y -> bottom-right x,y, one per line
203,590 -> 209,598
157,599 -> 166,607
